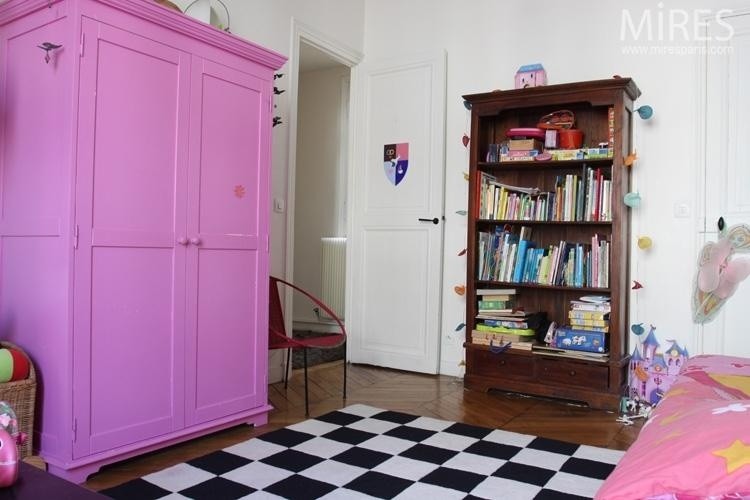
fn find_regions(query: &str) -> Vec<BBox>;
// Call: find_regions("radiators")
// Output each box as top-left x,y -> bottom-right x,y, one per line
316,236 -> 346,320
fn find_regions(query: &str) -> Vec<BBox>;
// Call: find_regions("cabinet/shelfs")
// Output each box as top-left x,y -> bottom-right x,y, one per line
1,1 -> 288,487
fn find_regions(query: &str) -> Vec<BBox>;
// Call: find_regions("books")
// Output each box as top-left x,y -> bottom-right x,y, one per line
470,106 -> 616,364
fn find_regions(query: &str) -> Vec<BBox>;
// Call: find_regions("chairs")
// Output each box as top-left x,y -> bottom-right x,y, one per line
268,275 -> 347,415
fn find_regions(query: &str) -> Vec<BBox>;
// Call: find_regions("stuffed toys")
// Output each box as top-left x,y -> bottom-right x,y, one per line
691,216 -> 749,326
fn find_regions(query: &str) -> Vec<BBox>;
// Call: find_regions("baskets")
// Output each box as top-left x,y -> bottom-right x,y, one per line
0,341 -> 37,461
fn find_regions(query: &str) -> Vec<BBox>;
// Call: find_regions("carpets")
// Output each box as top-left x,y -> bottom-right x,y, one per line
94,401 -> 628,500
